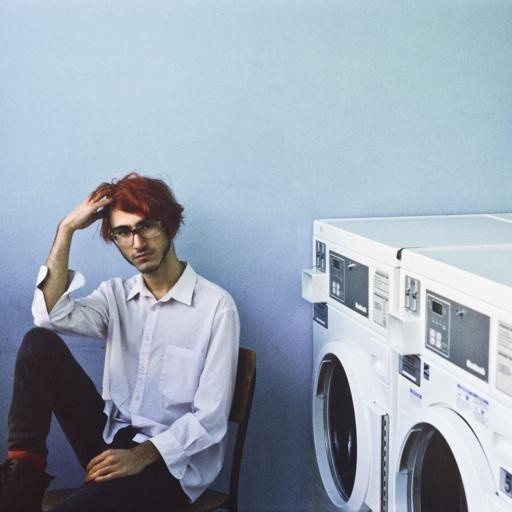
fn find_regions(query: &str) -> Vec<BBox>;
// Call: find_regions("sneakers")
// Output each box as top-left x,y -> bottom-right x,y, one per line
0,458 -> 55,512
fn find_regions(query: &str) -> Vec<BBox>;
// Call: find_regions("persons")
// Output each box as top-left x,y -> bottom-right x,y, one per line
0,168 -> 243,512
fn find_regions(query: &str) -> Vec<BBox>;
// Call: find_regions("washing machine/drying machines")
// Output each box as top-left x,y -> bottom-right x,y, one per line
382,244 -> 512,512
299,213 -> 512,512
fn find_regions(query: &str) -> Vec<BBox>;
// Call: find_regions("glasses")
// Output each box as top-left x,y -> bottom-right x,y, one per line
110,218 -> 162,246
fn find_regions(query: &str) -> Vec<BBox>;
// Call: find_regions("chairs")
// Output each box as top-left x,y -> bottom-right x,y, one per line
41,346 -> 257,512
